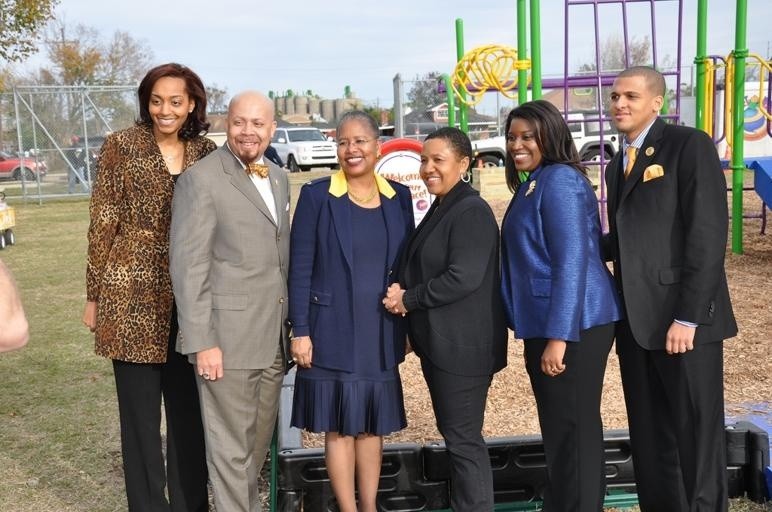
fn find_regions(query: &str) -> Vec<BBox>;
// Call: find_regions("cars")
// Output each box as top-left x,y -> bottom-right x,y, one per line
0,149 -> 48,182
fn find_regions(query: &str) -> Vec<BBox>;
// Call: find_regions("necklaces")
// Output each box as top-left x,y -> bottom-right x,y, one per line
348,184 -> 378,205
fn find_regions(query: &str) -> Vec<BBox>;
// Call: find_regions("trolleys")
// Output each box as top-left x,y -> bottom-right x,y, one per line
0,192 -> 17,251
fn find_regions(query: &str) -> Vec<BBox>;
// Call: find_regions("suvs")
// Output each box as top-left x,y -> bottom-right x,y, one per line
64,135 -> 107,183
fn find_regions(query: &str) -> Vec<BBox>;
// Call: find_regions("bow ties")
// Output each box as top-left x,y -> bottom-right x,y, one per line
244,162 -> 272,178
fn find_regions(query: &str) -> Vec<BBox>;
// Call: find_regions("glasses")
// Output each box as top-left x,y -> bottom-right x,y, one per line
336,136 -> 378,146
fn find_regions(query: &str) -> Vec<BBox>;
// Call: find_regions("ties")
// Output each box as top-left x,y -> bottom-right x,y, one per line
622,146 -> 639,179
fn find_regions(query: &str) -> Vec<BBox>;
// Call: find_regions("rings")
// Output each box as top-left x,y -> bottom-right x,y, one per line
202,373 -> 209,378
551,367 -> 556,372
394,307 -> 399,314
293,357 -> 298,361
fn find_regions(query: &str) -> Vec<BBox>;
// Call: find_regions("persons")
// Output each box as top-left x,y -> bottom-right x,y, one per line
169,93 -> 291,510
601,67 -> 738,512
382,127 -> 508,510
498,101 -> 621,511
284,112 -> 415,512
83,64 -> 219,512
0,259 -> 28,353
